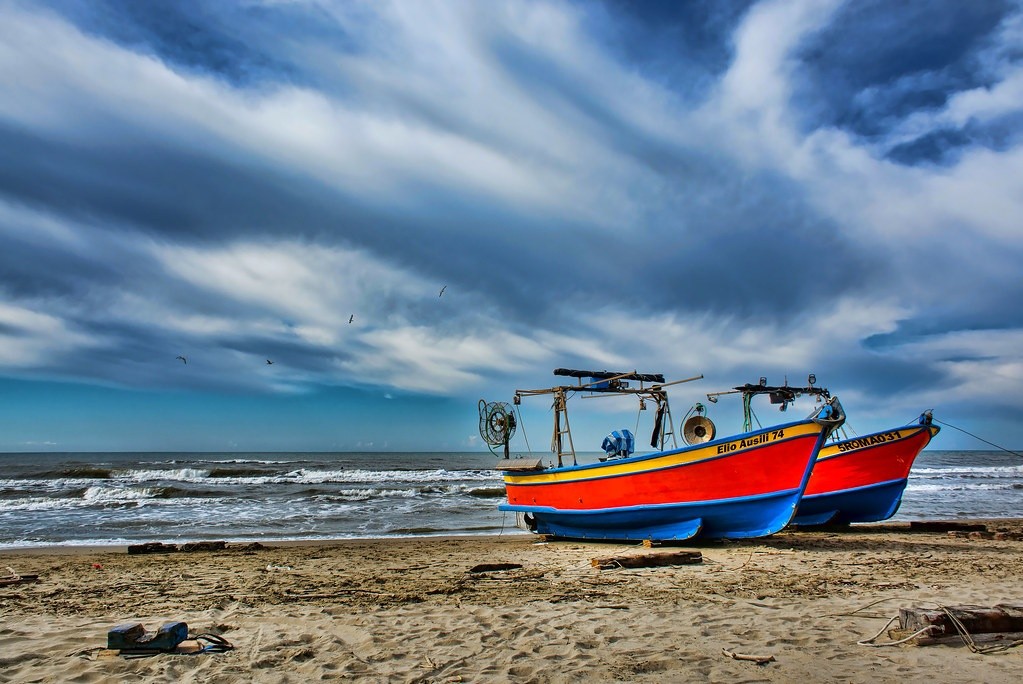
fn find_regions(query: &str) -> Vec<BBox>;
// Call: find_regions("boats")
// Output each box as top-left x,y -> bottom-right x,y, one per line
597,373 -> 941,528
478,369 -> 846,542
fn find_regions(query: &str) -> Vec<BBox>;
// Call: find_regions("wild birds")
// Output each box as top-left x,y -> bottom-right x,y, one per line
174,286 -> 444,365
